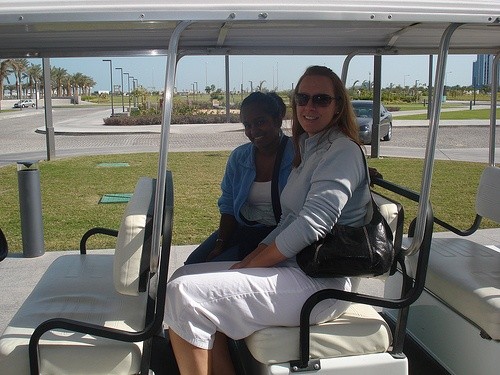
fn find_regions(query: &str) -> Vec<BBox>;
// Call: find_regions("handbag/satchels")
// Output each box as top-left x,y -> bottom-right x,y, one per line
296,223 -> 394,279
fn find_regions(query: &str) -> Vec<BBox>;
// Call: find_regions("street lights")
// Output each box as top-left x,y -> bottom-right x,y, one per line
115,67 -> 124,113
129,77 -> 139,107
102,59 -> 114,118
123,72 -> 130,107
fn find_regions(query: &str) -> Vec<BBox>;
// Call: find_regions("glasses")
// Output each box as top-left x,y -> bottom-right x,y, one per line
294,92 -> 338,108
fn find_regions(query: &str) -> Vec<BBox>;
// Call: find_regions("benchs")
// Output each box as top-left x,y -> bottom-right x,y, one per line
0,165 -> 500,375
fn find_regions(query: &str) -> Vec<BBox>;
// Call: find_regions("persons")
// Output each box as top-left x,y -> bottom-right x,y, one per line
183,91 -> 381,263
165,66 -> 372,375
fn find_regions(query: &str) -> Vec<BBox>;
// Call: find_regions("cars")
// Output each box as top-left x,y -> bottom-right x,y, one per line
349,99 -> 393,146
14,99 -> 35,108
